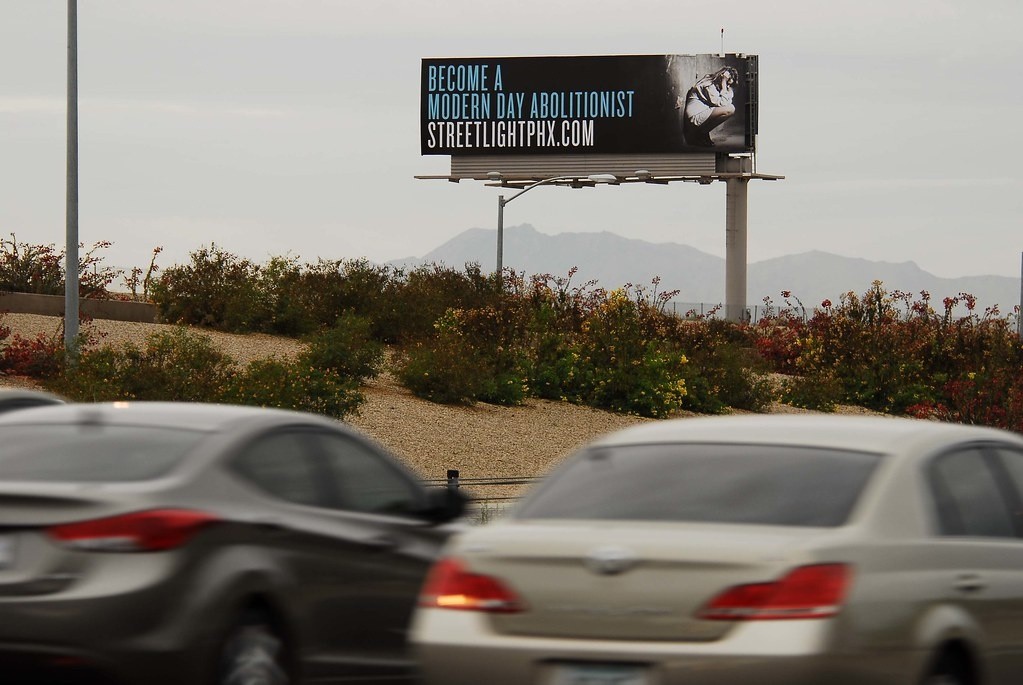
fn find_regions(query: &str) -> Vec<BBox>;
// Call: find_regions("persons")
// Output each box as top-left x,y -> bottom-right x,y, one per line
684,66 -> 738,144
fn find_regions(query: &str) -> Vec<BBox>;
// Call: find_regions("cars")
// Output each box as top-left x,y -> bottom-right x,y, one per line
409,410 -> 1023,685
1,401 -> 484,685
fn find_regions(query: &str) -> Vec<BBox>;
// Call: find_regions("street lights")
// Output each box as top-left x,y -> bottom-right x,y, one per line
498,173 -> 619,277
